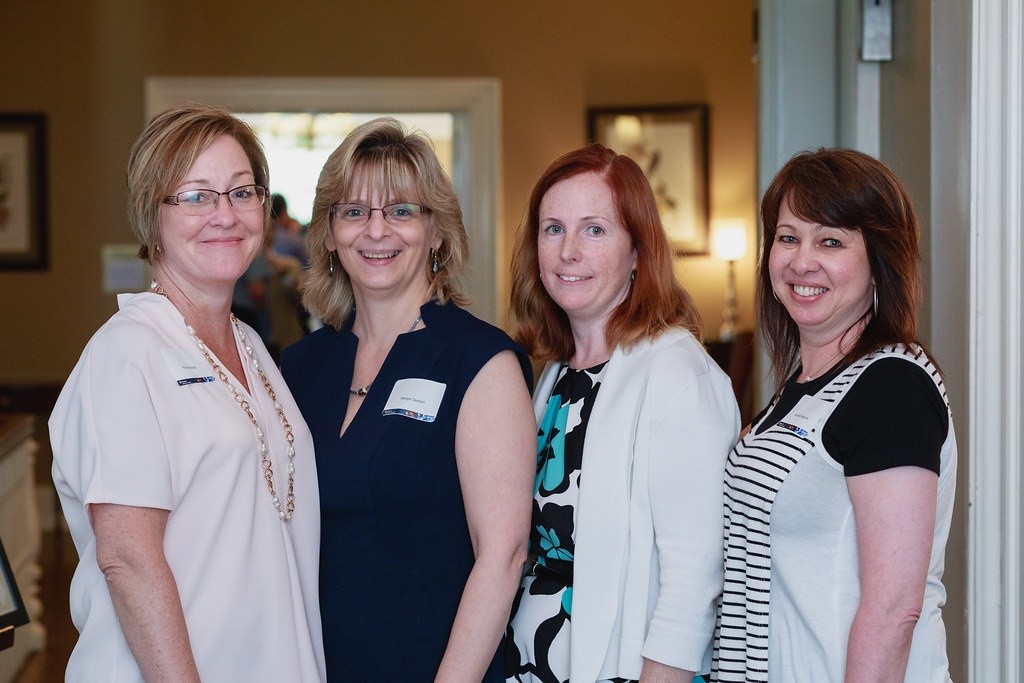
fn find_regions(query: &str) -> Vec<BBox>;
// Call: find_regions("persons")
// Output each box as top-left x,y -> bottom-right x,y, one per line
47,104 -> 537,683
505,143 -> 741,683
709,145 -> 957,683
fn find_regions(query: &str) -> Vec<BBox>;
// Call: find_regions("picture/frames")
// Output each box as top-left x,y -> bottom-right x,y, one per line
0,110 -> 51,271
585,102 -> 713,257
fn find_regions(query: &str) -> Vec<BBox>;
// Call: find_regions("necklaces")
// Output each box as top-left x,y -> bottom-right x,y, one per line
350,315 -> 422,396
797,331 -> 863,382
151,279 -> 299,522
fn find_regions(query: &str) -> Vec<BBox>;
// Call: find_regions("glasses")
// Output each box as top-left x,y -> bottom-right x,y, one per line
162,184 -> 269,215
331,202 -> 430,226
